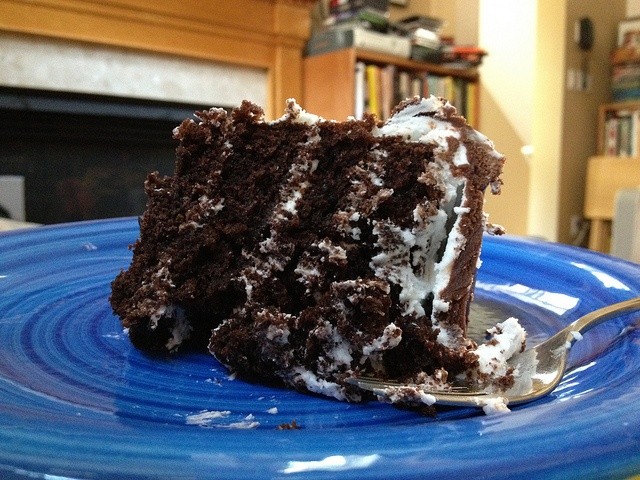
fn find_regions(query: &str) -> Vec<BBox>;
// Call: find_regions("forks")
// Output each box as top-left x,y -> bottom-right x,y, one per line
314,297 -> 640,409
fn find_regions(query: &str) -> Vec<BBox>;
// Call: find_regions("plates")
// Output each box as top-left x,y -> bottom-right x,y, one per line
1,213 -> 638,480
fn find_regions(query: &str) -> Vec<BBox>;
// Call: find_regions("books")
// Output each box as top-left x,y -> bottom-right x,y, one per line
600,106 -> 639,158
355,59 -> 479,135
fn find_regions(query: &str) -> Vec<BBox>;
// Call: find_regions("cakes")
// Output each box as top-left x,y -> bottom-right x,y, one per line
108,93 -> 528,421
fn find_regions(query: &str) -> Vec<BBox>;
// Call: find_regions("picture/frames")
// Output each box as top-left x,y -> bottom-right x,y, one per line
616,17 -> 640,47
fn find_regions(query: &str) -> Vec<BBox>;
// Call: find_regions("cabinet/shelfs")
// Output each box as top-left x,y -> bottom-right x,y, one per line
302,46 -> 478,135
583,101 -> 640,261
